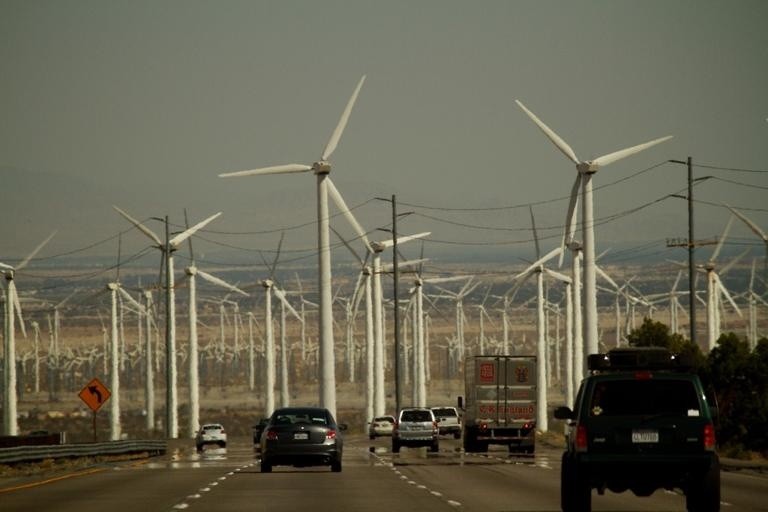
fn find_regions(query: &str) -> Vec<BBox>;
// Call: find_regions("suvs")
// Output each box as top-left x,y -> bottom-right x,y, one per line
548,344 -> 722,512
430,405 -> 462,440
389,405 -> 442,454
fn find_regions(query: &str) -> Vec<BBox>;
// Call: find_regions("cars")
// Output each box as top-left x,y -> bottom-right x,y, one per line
366,414 -> 397,441
191,423 -> 227,451
251,417 -> 270,459
188,448 -> 229,461
257,404 -> 350,473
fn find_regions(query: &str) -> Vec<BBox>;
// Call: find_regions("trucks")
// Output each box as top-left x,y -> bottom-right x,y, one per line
455,353 -> 541,457
